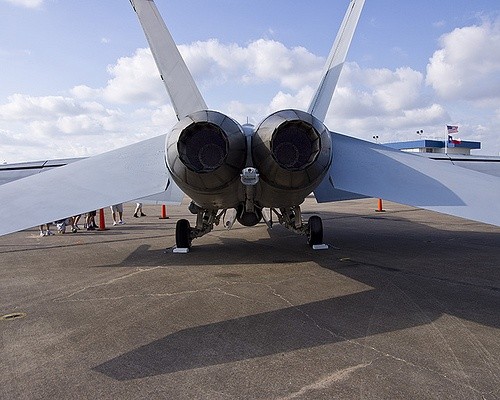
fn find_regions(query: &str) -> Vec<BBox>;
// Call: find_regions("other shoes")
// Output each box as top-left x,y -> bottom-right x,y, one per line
119,221 -> 124,224
72,228 -> 76,232
114,221 -> 116,225
93,225 -> 98,228
46,231 -> 54,235
134,215 -> 138,217
141,213 -> 146,216
39,232 -> 45,236
87,226 -> 95,230
74,224 -> 80,230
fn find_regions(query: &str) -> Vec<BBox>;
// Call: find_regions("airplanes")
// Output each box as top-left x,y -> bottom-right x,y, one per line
0,0 -> 500,253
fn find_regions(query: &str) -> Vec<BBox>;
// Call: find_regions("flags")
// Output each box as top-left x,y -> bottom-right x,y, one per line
447,126 -> 458,133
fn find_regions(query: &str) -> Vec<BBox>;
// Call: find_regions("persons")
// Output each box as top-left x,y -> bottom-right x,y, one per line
39,224 -> 54,236
84,210 -> 99,230
57,215 -> 82,234
133,203 -> 146,217
110,204 -> 124,226
